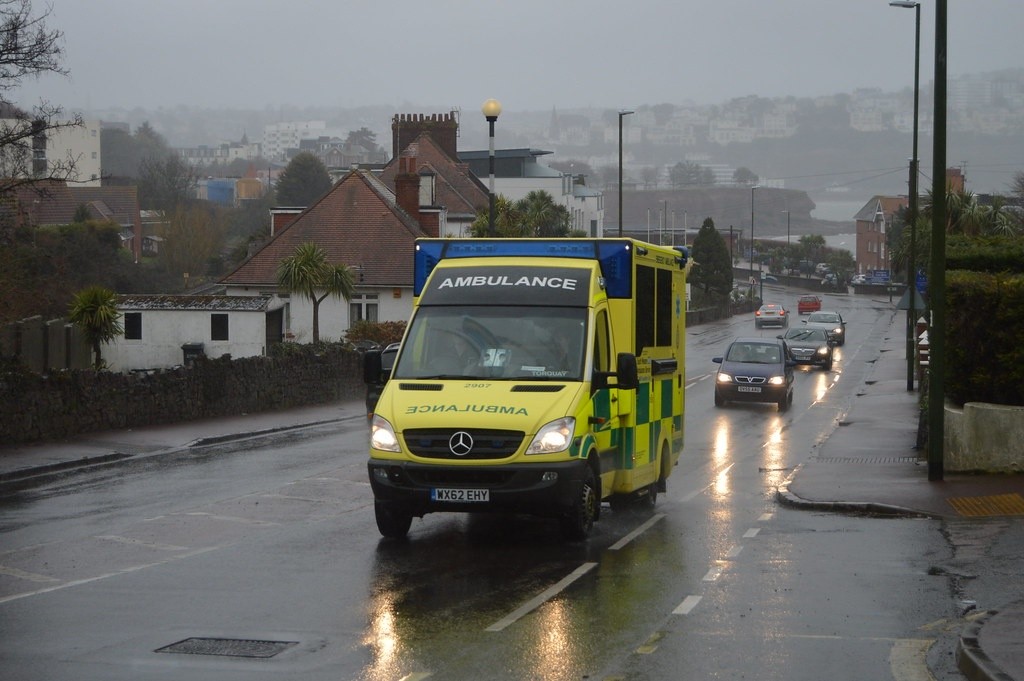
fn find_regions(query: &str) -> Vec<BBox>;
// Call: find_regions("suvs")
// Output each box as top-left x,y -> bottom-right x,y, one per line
365,342 -> 401,424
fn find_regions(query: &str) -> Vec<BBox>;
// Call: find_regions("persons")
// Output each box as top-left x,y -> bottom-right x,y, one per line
450,329 -> 485,369
548,331 -> 579,376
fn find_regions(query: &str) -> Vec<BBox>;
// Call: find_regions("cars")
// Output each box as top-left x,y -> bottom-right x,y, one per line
799,260 -> 817,273
815,263 -> 831,277
777,325 -> 835,371
851,275 -> 865,286
782,269 -> 800,277
825,274 -> 847,284
801,311 -> 847,346
755,304 -> 790,329
797,295 -> 822,315
712,337 -> 797,410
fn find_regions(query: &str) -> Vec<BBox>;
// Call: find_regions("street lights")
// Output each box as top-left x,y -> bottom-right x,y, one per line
751,186 -> 760,276
482,99 -> 502,238
876,212 -> 894,302
782,211 -> 790,286
907,158 -> 921,207
890,0 -> 920,392
619,110 -> 635,238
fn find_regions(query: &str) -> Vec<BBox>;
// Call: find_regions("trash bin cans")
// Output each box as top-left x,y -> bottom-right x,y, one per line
181,341 -> 204,370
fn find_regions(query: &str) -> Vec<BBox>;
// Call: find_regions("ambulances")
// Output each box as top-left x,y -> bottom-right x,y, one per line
363,237 -> 689,540
